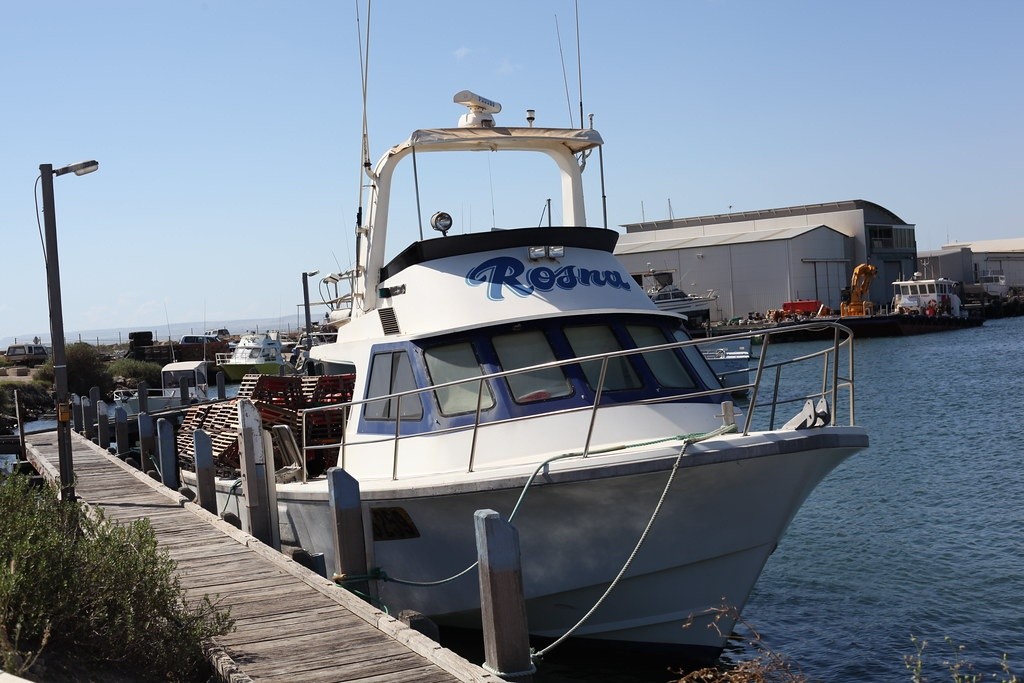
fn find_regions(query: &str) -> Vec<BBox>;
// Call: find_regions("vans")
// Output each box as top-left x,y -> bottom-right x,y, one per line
6,344 -> 49,364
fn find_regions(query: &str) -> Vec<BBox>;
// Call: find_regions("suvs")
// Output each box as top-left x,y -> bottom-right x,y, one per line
180,335 -> 236,352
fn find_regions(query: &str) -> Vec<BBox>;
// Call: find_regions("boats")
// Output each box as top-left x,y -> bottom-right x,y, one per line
777,257 -> 986,341
216,333 -> 296,381
963,274 -> 1009,300
637,263 -> 716,335
178,0 -> 872,651
113,307 -> 218,415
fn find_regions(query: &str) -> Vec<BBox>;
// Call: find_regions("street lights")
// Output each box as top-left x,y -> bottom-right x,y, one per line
39,159 -> 101,538
302,269 -> 321,376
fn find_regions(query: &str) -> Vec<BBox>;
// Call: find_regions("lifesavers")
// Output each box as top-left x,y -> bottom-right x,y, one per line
928,299 -> 937,307
